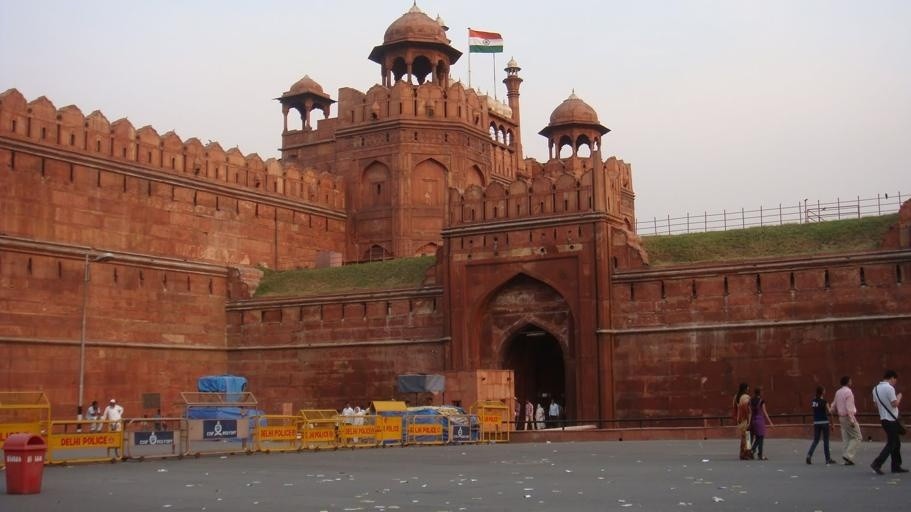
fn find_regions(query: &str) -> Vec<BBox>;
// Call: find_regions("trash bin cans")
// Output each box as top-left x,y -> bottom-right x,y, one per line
0,433 -> 48,494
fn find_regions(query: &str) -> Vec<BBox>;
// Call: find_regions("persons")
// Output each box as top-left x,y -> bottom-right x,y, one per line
549,399 -> 559,428
747,386 -> 775,460
733,383 -> 753,460
152,409 -> 166,431
427,398 -> 433,406
535,404 -> 546,430
524,398 -> 535,430
806,385 -> 837,465
103,400 -> 124,432
829,377 -> 862,466
869,370 -> 909,475
514,396 -> 520,429
86,401 -> 103,431
342,401 -> 372,425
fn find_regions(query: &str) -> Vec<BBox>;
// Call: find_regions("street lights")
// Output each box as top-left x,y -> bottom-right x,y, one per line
74,250 -> 120,433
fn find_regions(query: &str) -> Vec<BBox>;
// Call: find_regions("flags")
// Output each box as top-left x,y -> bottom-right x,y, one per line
468,29 -> 503,53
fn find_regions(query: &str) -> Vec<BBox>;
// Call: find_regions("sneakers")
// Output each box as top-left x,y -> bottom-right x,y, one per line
826,458 -> 837,464
841,454 -> 855,466
806,455 -> 811,464
891,467 -> 911,474
870,462 -> 886,475
747,449 -> 768,460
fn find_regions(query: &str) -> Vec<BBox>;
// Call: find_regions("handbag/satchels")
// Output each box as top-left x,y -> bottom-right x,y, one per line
894,417 -> 907,436
